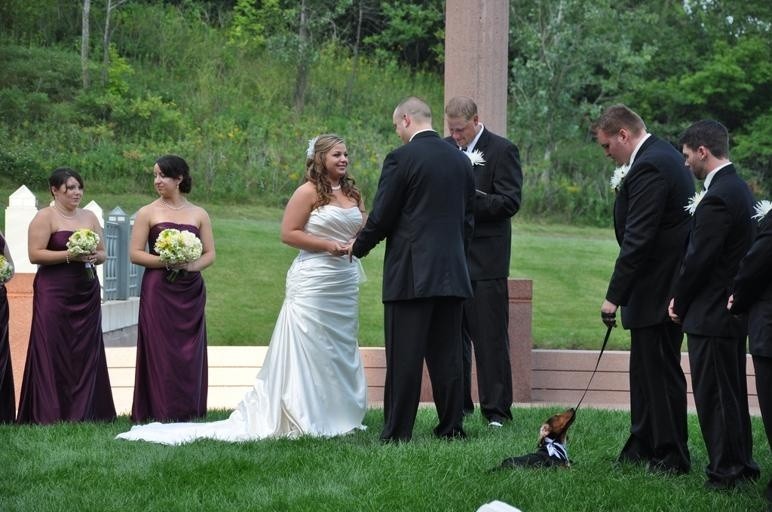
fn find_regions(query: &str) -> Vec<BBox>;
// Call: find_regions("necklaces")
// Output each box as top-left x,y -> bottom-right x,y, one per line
56,207 -> 77,219
161,199 -> 186,210
331,183 -> 341,191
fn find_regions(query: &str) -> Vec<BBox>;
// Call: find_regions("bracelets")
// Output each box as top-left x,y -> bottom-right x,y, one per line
66,252 -> 70,264
165,260 -> 170,272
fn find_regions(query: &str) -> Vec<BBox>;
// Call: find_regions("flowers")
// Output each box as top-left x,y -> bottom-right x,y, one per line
66,229 -> 101,281
0,254 -> 14,283
153,228 -> 203,282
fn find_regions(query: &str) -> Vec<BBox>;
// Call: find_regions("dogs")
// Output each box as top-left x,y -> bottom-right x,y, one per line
481,407 -> 577,476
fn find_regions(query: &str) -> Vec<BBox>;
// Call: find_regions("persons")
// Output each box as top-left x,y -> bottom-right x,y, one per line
668,118 -> 761,491
17,168 -> 117,424
727,200 -> 772,503
114,135 -> 370,444
128,155 -> 218,422
439,96 -> 523,430
343,96 -> 476,445
596,103 -> 696,478
0,232 -> 15,424
